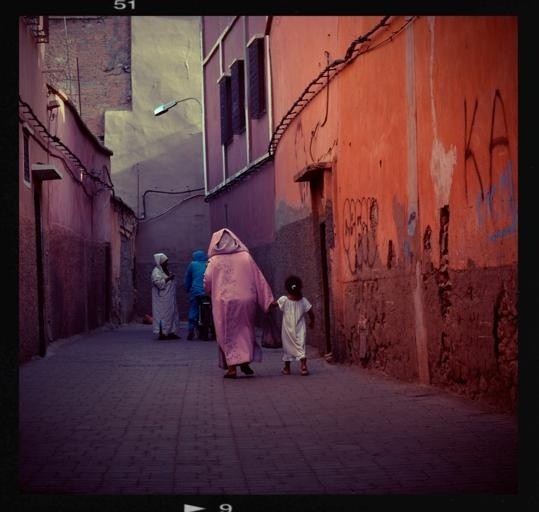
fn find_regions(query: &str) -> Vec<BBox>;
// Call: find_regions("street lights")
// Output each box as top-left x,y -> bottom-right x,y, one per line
154,97 -> 202,117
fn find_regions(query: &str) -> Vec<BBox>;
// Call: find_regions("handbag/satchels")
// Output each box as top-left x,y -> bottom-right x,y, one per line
262,313 -> 282,348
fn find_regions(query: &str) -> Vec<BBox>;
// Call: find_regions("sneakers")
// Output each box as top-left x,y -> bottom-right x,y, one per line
158,333 -> 180,339
187,332 -> 195,340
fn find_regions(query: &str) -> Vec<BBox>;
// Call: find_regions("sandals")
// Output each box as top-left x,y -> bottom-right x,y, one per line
240,364 -> 253,374
224,370 -> 236,378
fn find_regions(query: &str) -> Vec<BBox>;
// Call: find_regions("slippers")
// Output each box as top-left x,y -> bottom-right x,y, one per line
301,370 -> 309,375
280,367 -> 290,374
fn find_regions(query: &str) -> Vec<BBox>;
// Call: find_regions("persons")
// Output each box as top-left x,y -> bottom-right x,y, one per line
182,249 -> 209,340
203,228 -> 274,377
151,253 -> 184,340
272,276 -> 315,376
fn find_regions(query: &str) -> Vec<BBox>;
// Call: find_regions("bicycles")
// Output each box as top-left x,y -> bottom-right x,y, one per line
197,295 -> 213,340
